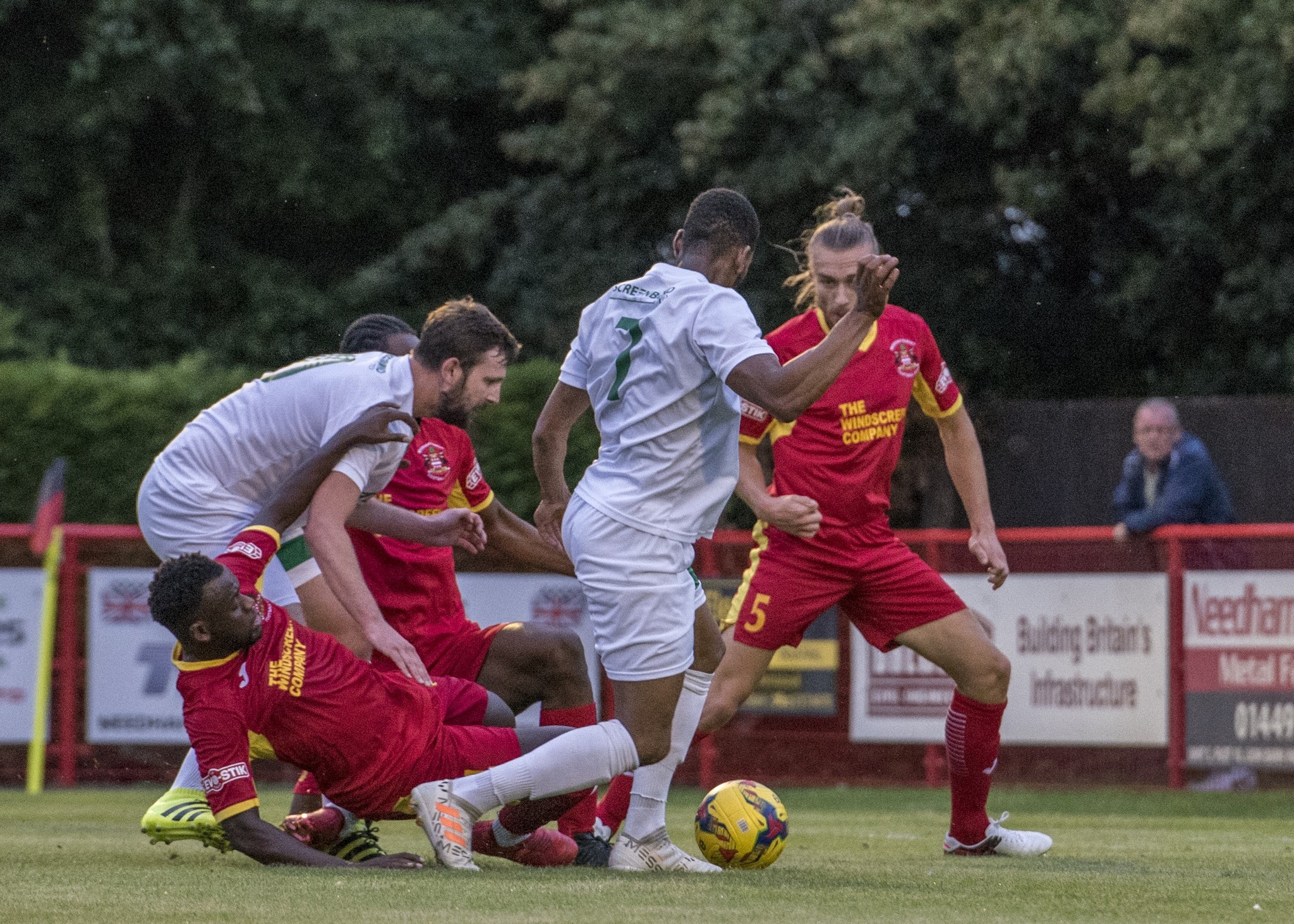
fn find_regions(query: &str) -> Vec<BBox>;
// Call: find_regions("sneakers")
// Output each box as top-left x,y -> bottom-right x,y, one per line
328,828 -> 386,862
279,809 -> 343,849
471,819 -> 578,867
944,811 -> 1054,856
410,778 -> 482,872
140,788 -> 237,853
572,831 -> 613,867
608,833 -> 723,874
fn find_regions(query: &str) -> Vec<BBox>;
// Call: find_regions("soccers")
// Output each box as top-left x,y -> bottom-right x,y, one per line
694,780 -> 789,870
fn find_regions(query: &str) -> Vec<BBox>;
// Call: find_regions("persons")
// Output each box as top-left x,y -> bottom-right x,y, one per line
289,313 -> 613,870
406,186 -> 901,873
134,296 -> 523,855
1109,397 -> 1264,796
149,400 -> 600,872
590,192 -> 1053,860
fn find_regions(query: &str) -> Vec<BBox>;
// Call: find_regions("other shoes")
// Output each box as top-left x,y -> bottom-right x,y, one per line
1195,770 -> 1258,789
